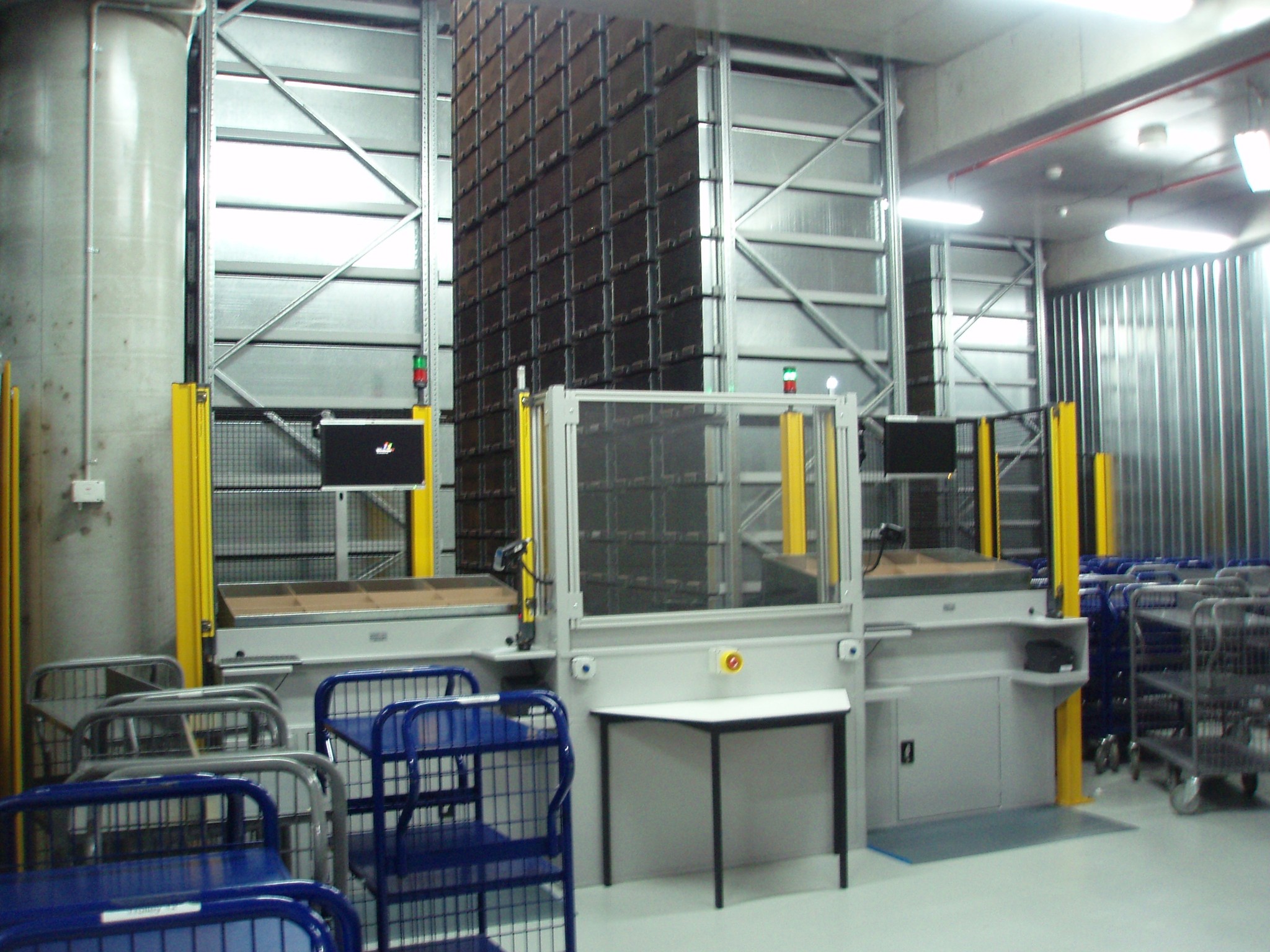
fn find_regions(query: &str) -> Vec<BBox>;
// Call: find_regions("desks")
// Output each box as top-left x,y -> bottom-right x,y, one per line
588,686 -> 853,909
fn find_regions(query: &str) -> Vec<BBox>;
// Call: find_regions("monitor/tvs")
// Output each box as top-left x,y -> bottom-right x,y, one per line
318,418 -> 425,490
884,413 -> 958,480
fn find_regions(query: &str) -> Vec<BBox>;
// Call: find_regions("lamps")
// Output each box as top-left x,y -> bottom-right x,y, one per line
1231,75 -> 1270,193
898,194 -> 985,228
1102,219 -> 1232,257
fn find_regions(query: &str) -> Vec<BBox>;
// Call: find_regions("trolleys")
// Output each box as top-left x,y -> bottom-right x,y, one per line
313,665 -> 578,952
1009,552 -> 1270,816
1,653 -> 364,952
